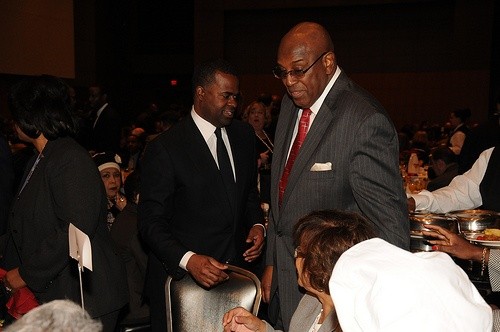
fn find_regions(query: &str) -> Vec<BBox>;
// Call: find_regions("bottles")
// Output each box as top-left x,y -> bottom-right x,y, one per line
408,153 -> 419,176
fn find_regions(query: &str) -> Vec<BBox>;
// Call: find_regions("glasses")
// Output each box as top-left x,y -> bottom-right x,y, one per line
294,246 -> 309,259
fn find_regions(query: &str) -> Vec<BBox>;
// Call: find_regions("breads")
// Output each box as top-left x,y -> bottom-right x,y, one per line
484,228 -> 500,241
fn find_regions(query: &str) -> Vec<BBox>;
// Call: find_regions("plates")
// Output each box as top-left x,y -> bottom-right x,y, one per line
466,233 -> 500,247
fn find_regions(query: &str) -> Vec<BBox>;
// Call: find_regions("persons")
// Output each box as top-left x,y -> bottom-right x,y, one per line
222,209 -> 380,332
397,106 -> 474,192
0,64 -> 190,230
406,95 -> 500,215
245,93 -> 280,202
0,73 -> 129,332
260,22 -> 410,332
136,63 -> 270,332
422,225 -> 500,332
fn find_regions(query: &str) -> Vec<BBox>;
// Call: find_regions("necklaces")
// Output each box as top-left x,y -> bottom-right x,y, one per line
255,127 -> 274,154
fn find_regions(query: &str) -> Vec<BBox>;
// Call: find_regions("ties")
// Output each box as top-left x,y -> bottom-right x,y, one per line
214,128 -> 236,194
279,108 -> 313,212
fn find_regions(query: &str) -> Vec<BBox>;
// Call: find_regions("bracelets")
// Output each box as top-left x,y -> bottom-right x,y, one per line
481,248 -> 487,276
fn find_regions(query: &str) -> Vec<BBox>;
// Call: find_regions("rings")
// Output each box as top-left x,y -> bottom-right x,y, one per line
6,287 -> 12,292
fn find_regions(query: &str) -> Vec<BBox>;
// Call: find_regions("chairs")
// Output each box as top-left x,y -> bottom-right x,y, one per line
165,264 -> 262,332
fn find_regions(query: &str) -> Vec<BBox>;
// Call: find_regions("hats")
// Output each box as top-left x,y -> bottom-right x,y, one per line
328,237 -> 493,332
273,50 -> 336,79
92,152 -> 120,172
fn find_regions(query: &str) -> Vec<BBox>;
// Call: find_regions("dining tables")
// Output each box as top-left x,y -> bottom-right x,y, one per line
410,210 -> 500,304
